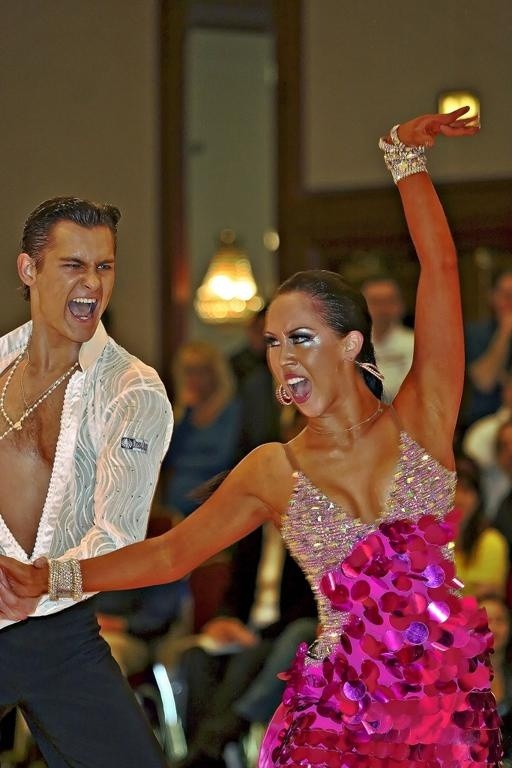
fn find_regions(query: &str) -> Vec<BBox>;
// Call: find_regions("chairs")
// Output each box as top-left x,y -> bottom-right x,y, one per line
130,662 -> 191,764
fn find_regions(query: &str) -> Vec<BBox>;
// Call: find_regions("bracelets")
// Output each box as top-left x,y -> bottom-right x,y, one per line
375,122 -> 431,185
49,559 -> 83,601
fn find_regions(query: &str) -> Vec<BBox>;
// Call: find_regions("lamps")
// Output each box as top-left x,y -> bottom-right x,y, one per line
438,86 -> 482,128
193,227 -> 264,325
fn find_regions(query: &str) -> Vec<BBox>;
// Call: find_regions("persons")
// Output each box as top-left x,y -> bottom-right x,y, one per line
229,448 -> 505,767
0,196 -> 181,768
178,520 -> 317,768
460,268 -> 510,533
1,196 -> 181,767
0,100 -> 504,768
359,272 -> 416,403
160,342 -> 245,629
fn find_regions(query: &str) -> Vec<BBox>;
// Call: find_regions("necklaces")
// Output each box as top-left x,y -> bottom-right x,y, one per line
0,345 -> 79,439
308,398 -> 382,433
